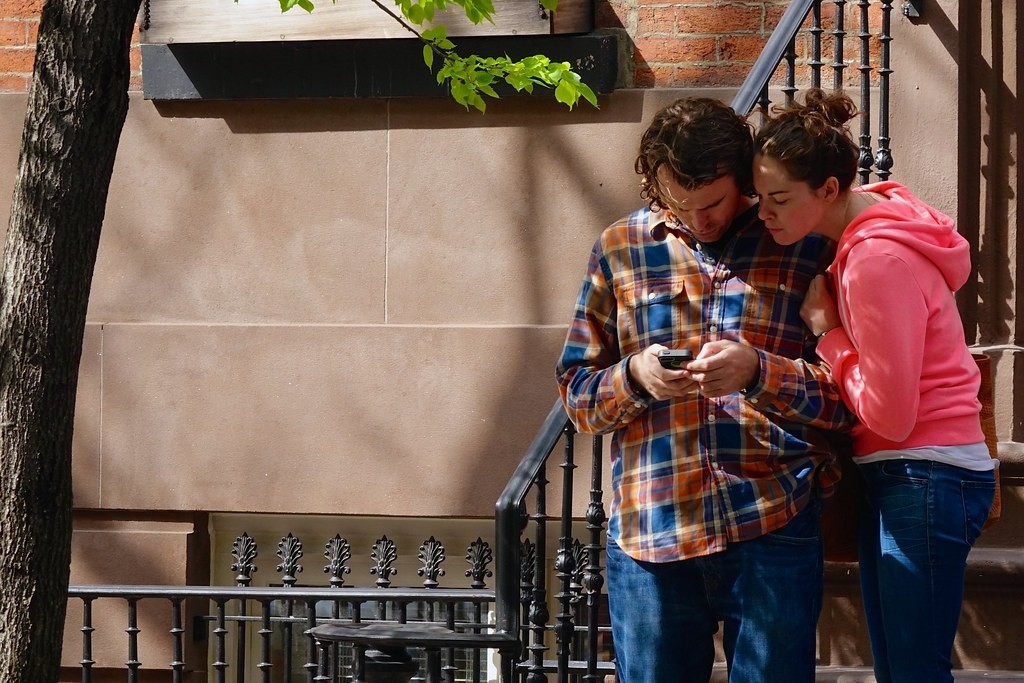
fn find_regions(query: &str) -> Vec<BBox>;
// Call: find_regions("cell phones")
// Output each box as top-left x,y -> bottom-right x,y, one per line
657,349 -> 694,381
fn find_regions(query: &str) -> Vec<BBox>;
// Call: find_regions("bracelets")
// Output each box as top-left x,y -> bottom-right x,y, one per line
816,331 -> 827,338
739,373 -> 759,396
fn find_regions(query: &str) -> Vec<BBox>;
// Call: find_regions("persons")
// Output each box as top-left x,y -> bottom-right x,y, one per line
751,91 -> 997,683
554,96 -> 857,683
